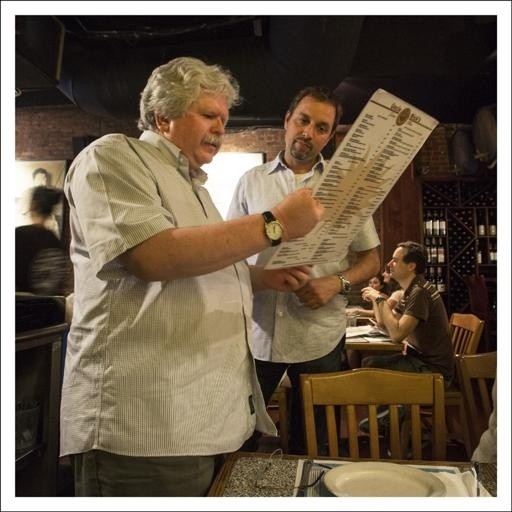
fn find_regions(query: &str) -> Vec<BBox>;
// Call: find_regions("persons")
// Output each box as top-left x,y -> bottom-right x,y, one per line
58,56 -> 325,496
347,240 -> 455,435
224,85 -> 383,456
13,167 -> 66,241
13,185 -> 70,329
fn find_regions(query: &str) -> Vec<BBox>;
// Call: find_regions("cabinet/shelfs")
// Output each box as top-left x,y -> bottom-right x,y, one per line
380,125 -> 496,345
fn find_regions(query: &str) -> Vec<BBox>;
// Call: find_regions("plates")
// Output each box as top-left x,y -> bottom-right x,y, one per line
323,462 -> 447,497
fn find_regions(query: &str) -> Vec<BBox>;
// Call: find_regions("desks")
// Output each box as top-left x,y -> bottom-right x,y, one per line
342,323 -> 406,367
209,448 -> 498,496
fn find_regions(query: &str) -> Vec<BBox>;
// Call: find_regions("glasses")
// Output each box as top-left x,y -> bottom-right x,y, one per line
252,446 -> 331,491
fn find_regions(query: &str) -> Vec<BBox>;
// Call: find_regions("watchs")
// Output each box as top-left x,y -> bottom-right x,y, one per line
261,210 -> 285,248
335,271 -> 352,296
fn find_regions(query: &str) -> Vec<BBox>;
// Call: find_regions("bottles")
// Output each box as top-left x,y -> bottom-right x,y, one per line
476,209 -> 497,265
423,208 -> 445,284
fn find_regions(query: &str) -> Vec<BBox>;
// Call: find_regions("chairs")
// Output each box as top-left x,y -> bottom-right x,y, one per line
300,367 -> 449,461
16,322 -> 69,473
454,350 -> 497,453
447,312 -> 484,359
